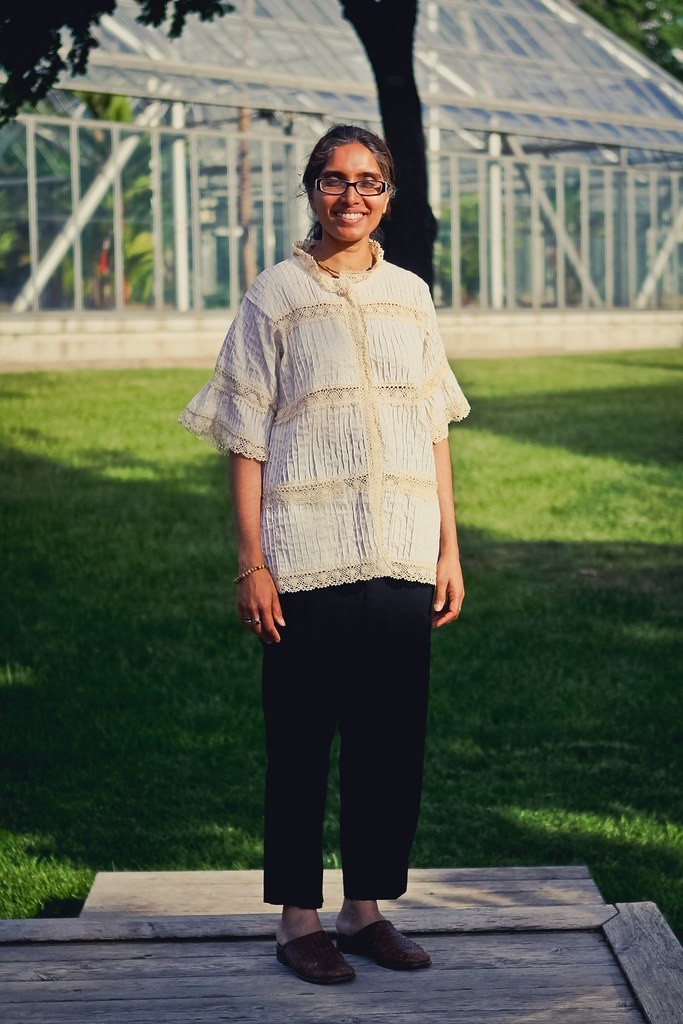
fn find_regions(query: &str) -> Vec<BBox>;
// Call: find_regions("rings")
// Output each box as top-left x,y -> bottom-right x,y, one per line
251,620 -> 261,625
239,617 -> 245,623
244,617 -> 251,623
459,608 -> 462,612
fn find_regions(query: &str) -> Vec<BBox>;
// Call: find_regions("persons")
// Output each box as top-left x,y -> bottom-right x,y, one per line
176,122 -> 470,985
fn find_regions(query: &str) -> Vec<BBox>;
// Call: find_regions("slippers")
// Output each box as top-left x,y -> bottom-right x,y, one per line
276,931 -> 356,985
336,920 -> 432,971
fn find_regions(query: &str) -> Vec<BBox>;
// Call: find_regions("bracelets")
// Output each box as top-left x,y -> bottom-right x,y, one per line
233,563 -> 269,583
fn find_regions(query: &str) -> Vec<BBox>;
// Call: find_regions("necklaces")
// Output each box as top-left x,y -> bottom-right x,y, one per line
310,249 -> 375,279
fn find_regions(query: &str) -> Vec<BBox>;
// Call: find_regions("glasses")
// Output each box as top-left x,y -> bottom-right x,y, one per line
314,177 -> 390,196
